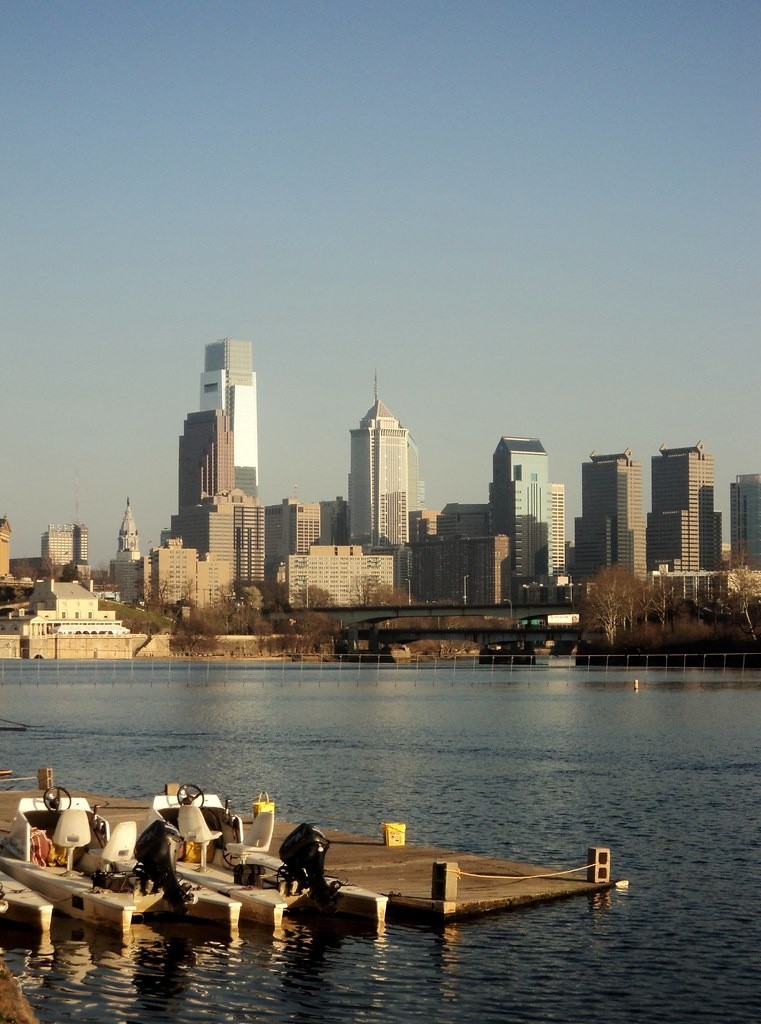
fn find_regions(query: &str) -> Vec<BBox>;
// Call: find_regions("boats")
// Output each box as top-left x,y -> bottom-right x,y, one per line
143,784 -> 391,934
1,872 -> 53,957
4,786 -> 244,939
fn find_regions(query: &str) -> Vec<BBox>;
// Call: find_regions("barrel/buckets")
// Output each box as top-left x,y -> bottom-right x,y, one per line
252,792 -> 274,819
381,822 -> 406,848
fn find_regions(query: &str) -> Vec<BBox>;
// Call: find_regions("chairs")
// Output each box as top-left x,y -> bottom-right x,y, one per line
226,811 -> 275,886
178,805 -> 223,874
52,808 -> 92,877
88,821 -> 136,873
